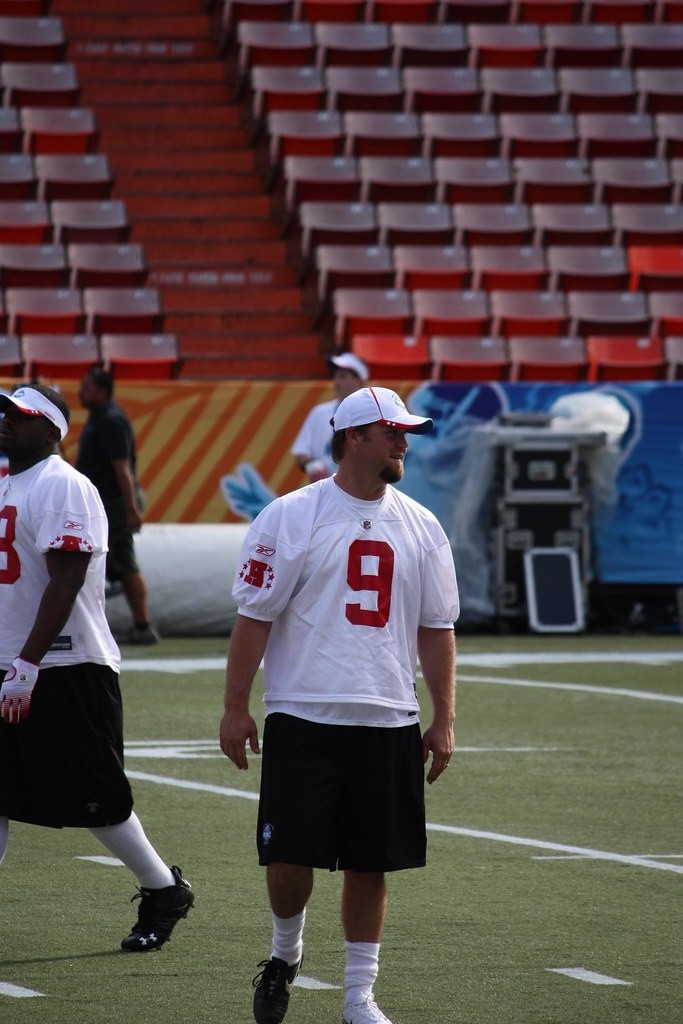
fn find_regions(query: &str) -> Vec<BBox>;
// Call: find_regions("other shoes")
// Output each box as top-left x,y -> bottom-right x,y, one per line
117,623 -> 158,645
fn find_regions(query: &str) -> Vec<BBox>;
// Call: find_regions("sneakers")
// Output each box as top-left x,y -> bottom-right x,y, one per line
253,950 -> 305,1024
120,865 -> 196,952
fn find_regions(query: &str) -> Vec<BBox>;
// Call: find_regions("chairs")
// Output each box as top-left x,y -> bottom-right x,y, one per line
0,16 -> 195,380
221,0 -> 682,381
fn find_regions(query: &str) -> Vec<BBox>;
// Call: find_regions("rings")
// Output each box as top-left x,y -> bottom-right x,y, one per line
446,764 -> 449,767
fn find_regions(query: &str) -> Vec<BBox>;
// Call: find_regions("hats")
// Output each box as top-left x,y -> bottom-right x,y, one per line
327,352 -> 369,386
333,385 -> 435,435
0,388 -> 68,442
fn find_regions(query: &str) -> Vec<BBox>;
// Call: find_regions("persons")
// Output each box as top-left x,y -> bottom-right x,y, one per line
0,369 -> 195,953
219,354 -> 461,1024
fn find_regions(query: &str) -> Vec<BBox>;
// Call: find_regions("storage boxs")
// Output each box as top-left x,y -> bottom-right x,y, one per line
493,444 -> 592,618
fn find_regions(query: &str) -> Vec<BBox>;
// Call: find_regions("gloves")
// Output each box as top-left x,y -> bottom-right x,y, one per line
0,655 -> 40,724
